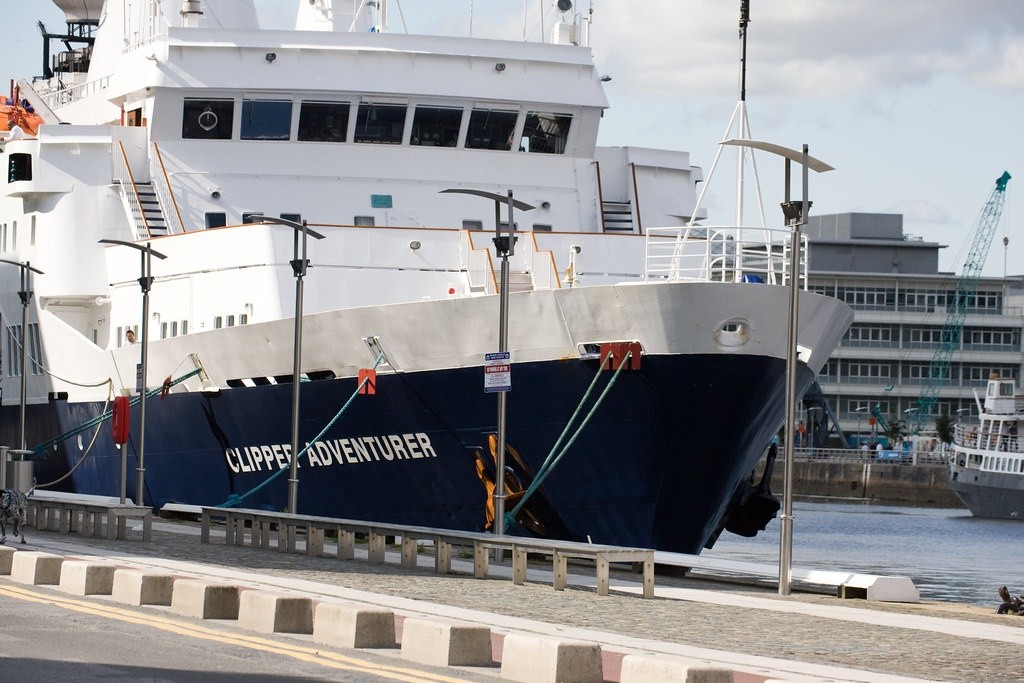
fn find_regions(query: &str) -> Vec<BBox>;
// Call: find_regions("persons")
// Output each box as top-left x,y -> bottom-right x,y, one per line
855,420 -> 1018,468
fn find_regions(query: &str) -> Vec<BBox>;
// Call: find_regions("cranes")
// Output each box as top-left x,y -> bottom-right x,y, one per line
877,170 -> 1012,465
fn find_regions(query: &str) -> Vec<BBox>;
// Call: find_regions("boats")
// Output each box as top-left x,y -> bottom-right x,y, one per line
1,1 -> 855,579
942,374 -> 1023,521
